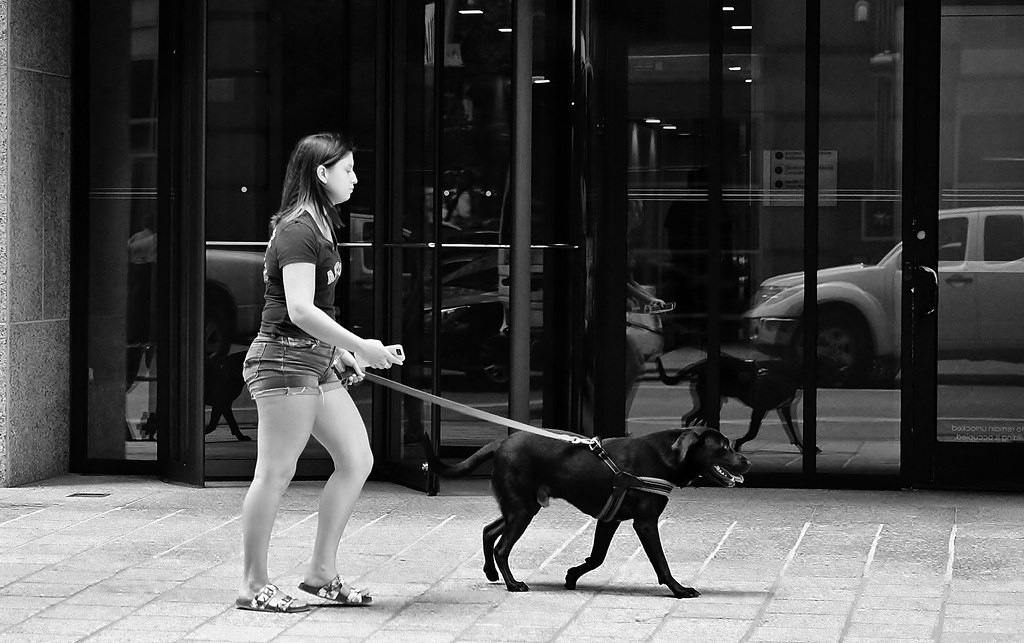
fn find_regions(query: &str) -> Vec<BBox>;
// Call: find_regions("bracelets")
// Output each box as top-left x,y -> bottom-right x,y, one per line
334,349 -> 345,360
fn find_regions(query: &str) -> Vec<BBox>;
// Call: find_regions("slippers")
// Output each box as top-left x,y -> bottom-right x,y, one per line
236,583 -> 310,613
299,575 -> 372,604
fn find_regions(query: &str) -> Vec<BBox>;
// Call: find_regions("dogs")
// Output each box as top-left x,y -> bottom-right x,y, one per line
424,426 -> 753,600
655,347 -> 824,456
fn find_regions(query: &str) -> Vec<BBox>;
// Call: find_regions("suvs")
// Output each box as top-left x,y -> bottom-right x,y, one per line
741,207 -> 1024,389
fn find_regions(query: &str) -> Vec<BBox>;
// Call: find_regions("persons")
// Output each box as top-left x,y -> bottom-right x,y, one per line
129,212 -> 156,442
236,133 -> 406,613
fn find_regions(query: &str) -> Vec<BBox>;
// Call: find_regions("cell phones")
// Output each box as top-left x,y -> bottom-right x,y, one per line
354,344 -> 405,368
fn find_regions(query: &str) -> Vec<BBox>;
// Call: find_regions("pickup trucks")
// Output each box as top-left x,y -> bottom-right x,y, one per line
202,213 -> 422,352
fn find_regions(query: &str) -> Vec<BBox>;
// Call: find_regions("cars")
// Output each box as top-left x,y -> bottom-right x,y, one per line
426,250 -> 667,400
